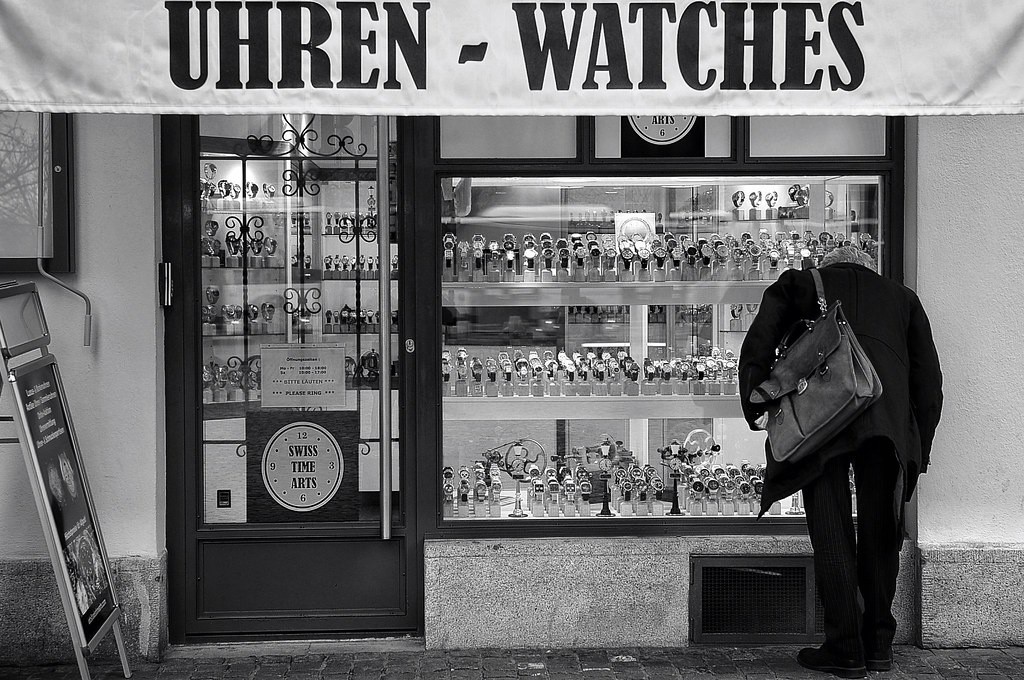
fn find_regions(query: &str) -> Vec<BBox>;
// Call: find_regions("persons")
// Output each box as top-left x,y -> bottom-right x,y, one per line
737,245 -> 945,679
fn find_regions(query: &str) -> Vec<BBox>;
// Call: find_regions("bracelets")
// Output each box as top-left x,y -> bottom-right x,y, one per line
618,218 -> 652,243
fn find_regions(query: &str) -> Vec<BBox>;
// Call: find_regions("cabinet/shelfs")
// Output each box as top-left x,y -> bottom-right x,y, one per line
439,279 -> 858,522
199,132 -> 398,419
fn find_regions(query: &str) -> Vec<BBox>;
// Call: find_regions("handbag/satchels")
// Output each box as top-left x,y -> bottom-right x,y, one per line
749,268 -> 883,463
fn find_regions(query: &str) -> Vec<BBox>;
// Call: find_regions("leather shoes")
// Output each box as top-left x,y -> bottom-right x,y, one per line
797,644 -> 866,678
865,648 -> 893,670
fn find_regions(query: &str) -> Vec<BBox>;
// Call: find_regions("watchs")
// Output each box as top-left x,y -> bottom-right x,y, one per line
44,451 -> 108,618
201,283 -> 276,324
290,305 -> 399,325
443,228 -> 879,270
570,206 -> 665,223
200,220 -> 279,256
200,163 -> 277,200
442,345 -> 740,384
290,195 -> 399,270
566,305 -> 761,328
441,444 -> 861,518
729,183 -> 834,208
202,363 -> 261,391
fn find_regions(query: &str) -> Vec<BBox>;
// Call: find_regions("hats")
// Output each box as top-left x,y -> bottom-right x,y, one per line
821,246 -> 877,271
